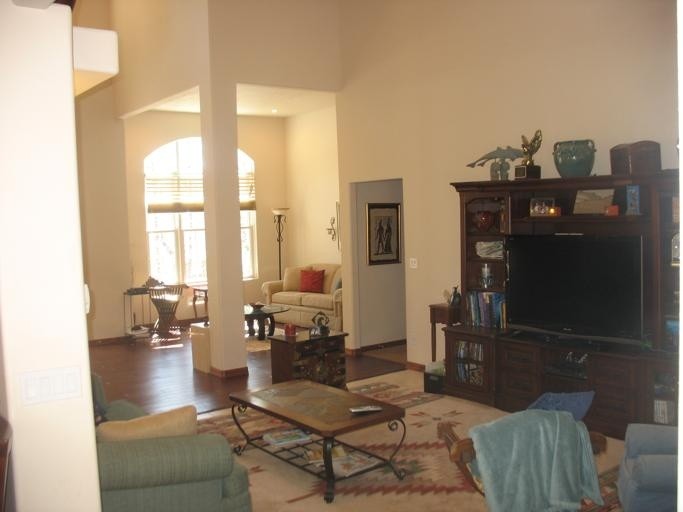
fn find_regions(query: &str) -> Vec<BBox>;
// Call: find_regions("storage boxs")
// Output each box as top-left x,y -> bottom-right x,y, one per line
424,369 -> 445,393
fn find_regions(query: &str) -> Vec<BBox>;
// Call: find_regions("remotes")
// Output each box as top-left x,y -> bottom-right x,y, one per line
348,403 -> 384,416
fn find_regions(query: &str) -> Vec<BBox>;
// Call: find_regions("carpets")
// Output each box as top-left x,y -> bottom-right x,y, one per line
197,369 -> 624,512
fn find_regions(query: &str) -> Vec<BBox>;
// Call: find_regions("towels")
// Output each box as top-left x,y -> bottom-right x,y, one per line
465,410 -> 605,512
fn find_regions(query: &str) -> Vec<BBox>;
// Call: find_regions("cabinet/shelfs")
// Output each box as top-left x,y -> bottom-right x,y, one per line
190,323 -> 211,374
266,327 -> 350,391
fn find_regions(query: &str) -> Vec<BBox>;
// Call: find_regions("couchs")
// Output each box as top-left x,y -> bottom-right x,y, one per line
262,263 -> 343,333
93,373 -> 253,512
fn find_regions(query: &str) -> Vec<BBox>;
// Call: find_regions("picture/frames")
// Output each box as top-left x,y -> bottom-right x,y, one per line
365,203 -> 402,265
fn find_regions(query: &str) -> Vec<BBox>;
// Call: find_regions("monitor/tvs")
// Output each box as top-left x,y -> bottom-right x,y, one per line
502,230 -> 643,347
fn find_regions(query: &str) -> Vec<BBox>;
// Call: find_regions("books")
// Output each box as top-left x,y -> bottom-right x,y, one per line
261,429 -> 310,448
653,383 -> 676,425
464,291 -> 506,330
302,445 -> 378,479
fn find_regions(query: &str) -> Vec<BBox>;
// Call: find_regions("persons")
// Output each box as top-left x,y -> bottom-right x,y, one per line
373,218 -> 385,255
384,217 -> 393,254
534,201 -> 549,213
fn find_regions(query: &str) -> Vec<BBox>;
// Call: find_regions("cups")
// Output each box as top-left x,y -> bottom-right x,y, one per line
284,323 -> 296,339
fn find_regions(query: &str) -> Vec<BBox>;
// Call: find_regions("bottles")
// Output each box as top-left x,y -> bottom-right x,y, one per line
482,262 -> 490,289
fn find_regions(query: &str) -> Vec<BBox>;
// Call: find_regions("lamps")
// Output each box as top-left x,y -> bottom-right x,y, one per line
272,208 -> 290,281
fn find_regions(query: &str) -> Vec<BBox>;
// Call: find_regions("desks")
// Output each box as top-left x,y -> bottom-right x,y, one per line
429,302 -> 461,362
123,284 -> 186,346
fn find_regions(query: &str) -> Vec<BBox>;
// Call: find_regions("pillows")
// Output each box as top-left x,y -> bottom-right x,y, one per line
283,263 -> 342,294
96,405 -> 197,444
525,388 -> 595,420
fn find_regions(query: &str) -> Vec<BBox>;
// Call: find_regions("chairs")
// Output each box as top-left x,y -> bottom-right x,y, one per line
438,420 -> 608,494
618,423 -> 677,512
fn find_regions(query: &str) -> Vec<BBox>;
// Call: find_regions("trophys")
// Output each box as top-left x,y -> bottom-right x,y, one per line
514,129 -> 543,181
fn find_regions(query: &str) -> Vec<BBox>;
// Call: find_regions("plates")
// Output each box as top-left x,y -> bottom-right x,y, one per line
249,302 -> 265,309
